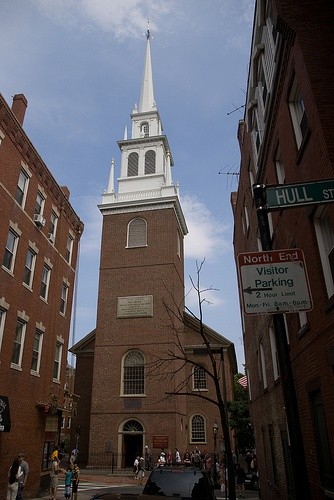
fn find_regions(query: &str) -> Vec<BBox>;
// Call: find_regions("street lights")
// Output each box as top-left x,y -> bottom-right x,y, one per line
211,419 -> 219,485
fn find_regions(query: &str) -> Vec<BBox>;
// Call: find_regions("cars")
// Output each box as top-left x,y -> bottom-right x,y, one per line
140,461 -> 221,500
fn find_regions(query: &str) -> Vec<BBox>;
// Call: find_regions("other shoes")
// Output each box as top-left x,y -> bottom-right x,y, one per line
239,493 -> 245,498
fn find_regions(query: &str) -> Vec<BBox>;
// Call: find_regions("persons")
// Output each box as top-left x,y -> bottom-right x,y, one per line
7,458 -> 24,500
71,460 -> 80,500
18,453 -> 29,500
70,446 -> 79,469
62,467 -> 73,500
133,445 -> 259,498
49,445 -> 63,500
